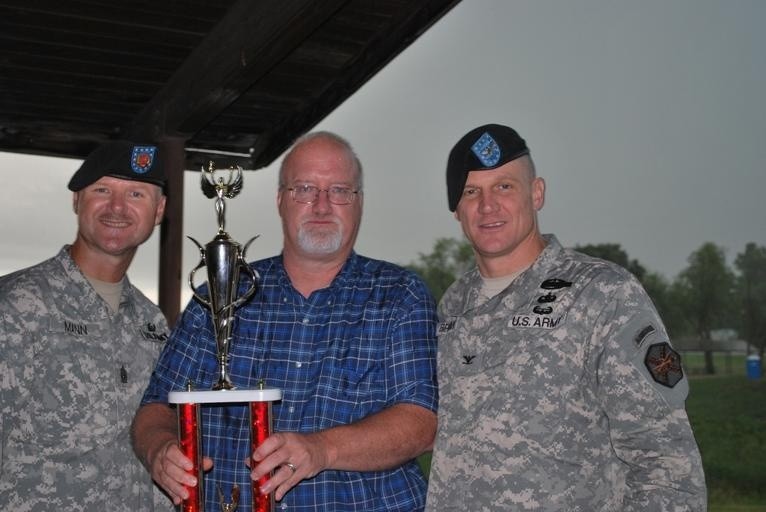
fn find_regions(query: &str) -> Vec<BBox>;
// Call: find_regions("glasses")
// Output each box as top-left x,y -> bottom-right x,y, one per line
283,185 -> 359,205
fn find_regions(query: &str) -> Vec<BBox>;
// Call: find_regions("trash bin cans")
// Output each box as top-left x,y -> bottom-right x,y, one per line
744,356 -> 762,381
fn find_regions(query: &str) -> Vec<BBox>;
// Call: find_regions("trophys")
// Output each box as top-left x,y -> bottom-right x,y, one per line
172,157 -> 283,510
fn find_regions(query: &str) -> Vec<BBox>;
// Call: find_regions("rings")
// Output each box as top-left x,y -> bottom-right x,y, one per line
278,460 -> 301,477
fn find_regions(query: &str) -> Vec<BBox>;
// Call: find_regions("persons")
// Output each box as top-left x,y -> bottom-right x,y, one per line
0,124 -> 190,511
417,121 -> 712,509
124,130 -> 440,510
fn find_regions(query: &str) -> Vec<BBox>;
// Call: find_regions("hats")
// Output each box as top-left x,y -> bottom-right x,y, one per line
69,140 -> 169,189
446,124 -> 529,211
